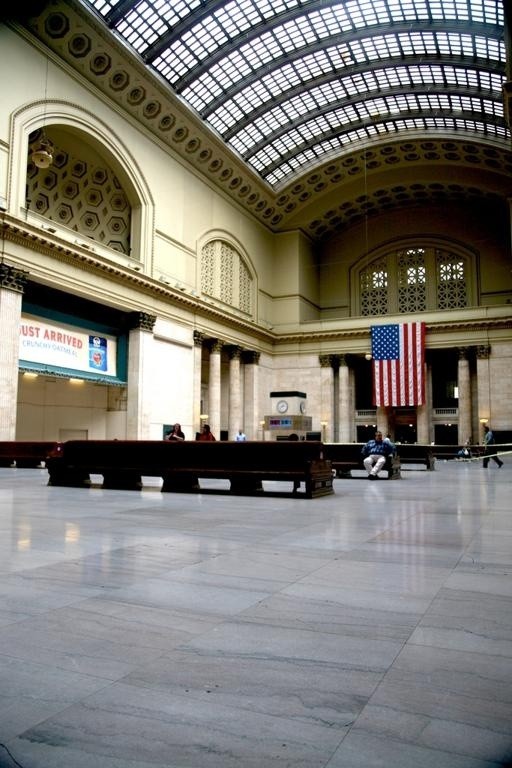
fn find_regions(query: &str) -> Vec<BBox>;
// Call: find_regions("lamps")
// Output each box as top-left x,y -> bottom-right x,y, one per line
26,0 -> 57,169
361,77 -> 379,362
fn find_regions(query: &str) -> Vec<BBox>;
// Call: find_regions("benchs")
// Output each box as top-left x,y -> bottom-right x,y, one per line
387,444 -> 487,469
0,439 -> 62,469
323,440 -> 401,480
56,431 -> 335,501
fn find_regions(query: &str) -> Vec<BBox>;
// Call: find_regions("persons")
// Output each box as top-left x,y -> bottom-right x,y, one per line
165,423 -> 185,441
288,434 -> 300,494
361,431 -> 396,479
234,429 -> 247,441
483,426 -> 503,468
384,434 -> 392,442
464,436 -> 472,444
199,425 -> 215,441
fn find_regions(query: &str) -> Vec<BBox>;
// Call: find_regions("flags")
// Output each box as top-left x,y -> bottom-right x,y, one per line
371,321 -> 425,407
